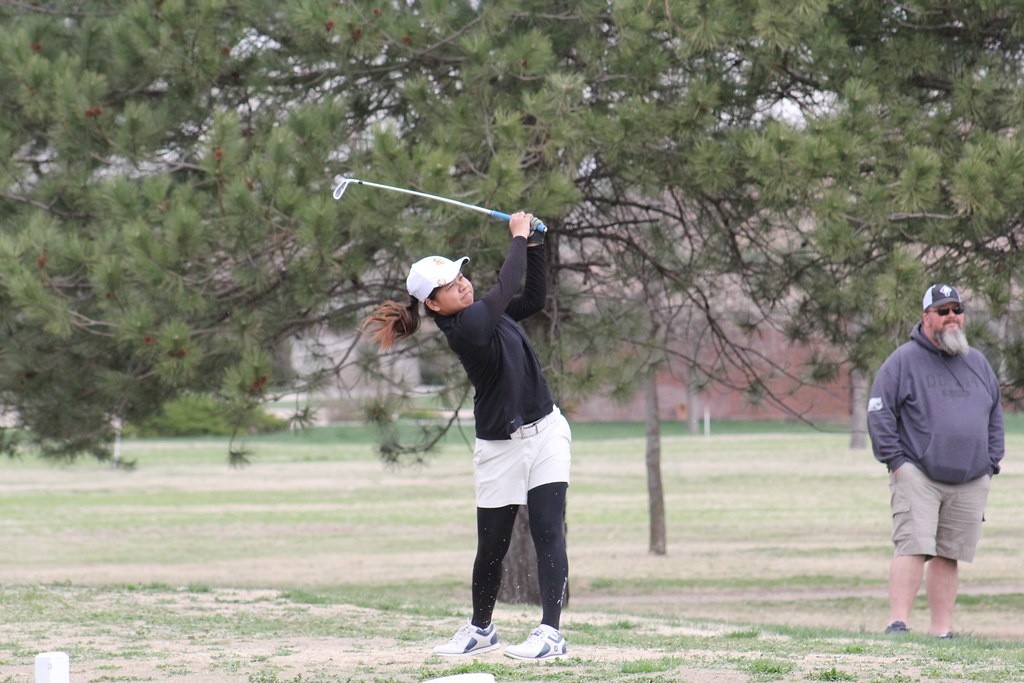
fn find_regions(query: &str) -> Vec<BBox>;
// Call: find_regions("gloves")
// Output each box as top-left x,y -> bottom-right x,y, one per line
526,217 -> 547,245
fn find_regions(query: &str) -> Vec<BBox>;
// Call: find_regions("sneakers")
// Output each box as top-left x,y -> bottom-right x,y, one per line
432,624 -> 501,658
504,628 -> 567,660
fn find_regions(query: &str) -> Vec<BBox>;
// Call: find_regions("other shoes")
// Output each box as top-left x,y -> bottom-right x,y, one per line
886,620 -> 912,634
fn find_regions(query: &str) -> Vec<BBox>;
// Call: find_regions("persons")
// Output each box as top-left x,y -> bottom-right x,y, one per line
866,284 -> 1003,641
364,211 -> 581,662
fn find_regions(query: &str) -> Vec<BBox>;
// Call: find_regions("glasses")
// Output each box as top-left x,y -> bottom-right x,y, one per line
926,305 -> 964,316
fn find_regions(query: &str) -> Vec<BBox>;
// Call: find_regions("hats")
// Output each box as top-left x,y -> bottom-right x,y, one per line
405,256 -> 470,304
922,284 -> 963,312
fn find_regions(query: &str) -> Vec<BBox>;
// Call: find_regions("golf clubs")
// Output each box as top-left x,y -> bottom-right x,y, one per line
331,170 -> 551,236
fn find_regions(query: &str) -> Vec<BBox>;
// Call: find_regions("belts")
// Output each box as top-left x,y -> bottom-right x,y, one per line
509,407 -> 560,439
